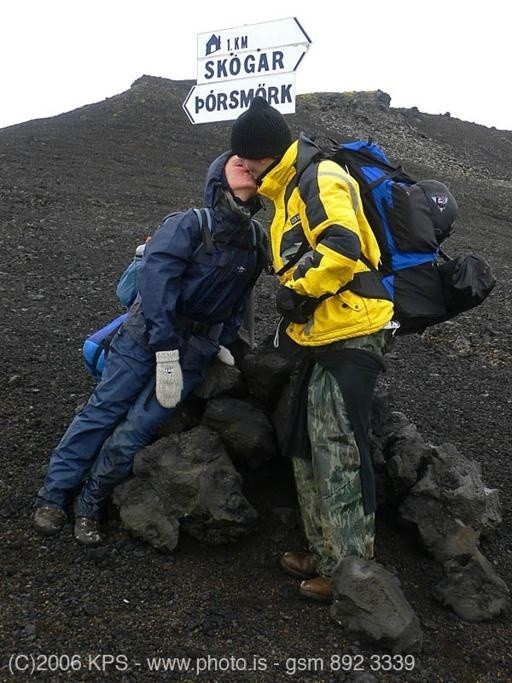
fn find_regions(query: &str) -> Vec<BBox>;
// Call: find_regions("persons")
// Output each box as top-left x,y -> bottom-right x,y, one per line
30,148 -> 278,547
227,90 -> 445,604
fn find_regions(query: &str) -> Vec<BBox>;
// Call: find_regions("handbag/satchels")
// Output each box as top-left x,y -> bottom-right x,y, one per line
437,252 -> 497,319
81,312 -> 131,381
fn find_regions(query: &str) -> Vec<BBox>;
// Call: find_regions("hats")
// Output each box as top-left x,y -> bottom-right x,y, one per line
230,95 -> 293,161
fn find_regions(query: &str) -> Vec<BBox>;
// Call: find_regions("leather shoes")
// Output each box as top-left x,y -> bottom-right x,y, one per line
299,575 -> 335,602
278,549 -> 323,579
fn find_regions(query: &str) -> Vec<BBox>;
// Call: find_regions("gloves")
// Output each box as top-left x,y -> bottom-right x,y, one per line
152,349 -> 185,411
216,343 -> 236,367
275,286 -> 311,324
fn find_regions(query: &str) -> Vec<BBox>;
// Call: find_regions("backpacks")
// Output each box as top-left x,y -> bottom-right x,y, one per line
115,208 -> 264,306
296,139 -> 451,335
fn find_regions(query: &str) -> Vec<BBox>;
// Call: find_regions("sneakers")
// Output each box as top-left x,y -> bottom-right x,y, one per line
34,501 -> 68,537
72,495 -> 101,549
